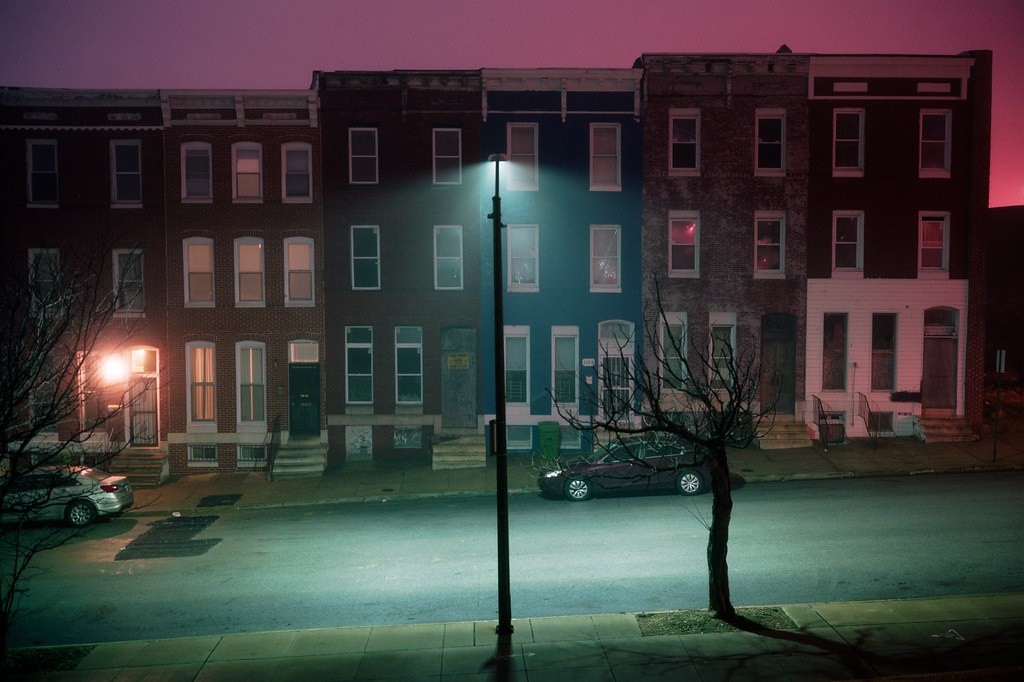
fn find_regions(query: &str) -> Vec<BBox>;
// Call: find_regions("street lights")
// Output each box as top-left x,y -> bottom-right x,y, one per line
486,154 -> 515,632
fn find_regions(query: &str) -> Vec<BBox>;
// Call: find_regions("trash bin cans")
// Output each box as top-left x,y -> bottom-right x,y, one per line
537,421 -> 561,459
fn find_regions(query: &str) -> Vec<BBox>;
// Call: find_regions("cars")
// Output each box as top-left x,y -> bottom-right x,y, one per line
536,437 -> 713,501
0,465 -> 135,528
